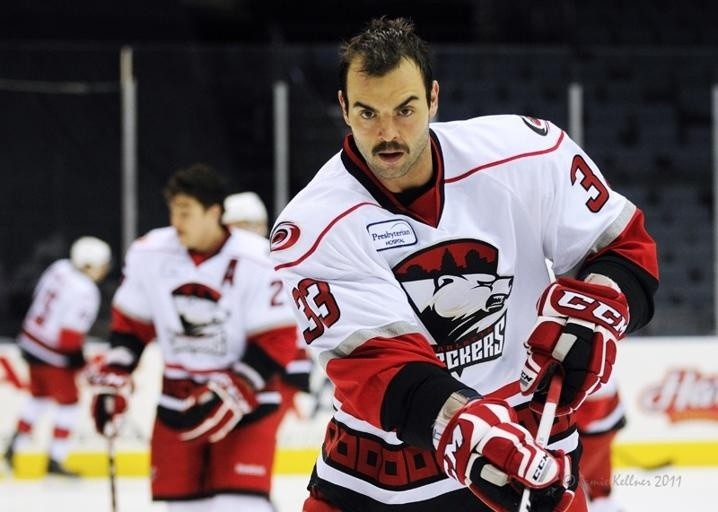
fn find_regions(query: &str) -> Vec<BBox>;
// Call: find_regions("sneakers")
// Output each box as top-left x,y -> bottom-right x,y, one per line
2,447 -> 15,470
48,461 -> 80,478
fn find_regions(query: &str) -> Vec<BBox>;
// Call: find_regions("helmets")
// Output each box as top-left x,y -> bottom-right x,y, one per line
70,236 -> 111,271
221,193 -> 268,223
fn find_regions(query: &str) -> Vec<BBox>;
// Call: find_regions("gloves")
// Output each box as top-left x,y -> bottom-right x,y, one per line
520,273 -> 630,417
89,367 -> 135,437
174,368 -> 261,444
431,388 -> 583,512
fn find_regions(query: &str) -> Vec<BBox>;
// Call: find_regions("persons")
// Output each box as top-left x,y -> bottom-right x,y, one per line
93,165 -> 311,512
571,370 -> 627,504
2,235 -> 110,481
261,14 -> 659,510
221,190 -> 271,240
